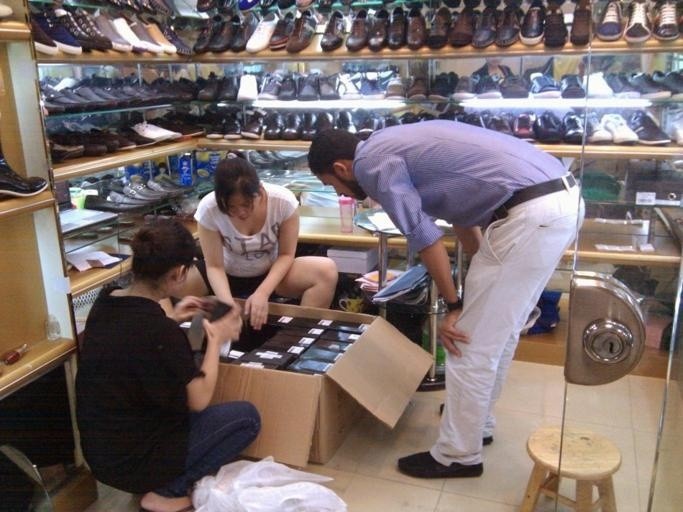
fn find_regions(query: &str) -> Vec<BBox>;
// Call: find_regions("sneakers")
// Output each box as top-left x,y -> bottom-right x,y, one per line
0,157 -> 48,197
0,3 -> 13,18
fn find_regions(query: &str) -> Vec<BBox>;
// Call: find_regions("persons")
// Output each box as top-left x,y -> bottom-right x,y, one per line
168,149 -> 339,332
308,119 -> 587,479
75,216 -> 262,512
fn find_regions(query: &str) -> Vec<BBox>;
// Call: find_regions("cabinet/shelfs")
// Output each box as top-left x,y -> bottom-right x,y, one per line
0,21 -> 82,511
33,34 -> 683,377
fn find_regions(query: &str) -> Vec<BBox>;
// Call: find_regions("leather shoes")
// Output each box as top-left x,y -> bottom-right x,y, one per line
440,403 -> 492,445
399,452 -> 483,478
80,150 -> 309,211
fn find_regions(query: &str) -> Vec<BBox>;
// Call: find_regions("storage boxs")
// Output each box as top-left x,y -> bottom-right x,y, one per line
186,295 -> 437,473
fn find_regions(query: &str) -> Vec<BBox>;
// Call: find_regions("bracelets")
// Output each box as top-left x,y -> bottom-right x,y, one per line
446,296 -> 463,311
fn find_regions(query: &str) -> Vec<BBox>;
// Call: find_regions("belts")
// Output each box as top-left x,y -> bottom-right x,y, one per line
489,174 -> 575,224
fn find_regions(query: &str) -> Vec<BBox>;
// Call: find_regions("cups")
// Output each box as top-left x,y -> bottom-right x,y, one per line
339,299 -> 363,313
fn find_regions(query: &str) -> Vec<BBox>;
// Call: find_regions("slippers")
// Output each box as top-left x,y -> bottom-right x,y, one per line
138,495 -> 196,512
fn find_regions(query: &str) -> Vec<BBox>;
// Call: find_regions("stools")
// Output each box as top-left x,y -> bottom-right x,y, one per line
517,426 -> 623,511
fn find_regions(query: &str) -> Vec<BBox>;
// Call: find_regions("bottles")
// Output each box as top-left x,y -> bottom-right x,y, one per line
421,307 -> 445,374
338,195 -> 354,233
